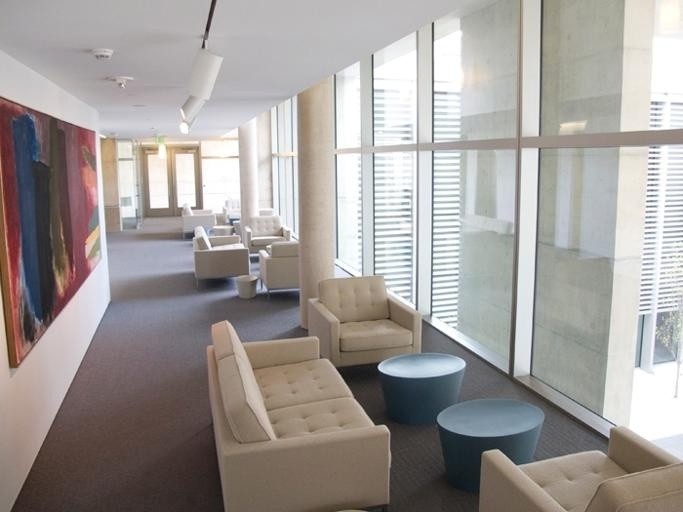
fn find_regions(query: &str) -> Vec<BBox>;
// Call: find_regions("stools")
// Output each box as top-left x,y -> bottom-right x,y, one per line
373,351 -> 545,490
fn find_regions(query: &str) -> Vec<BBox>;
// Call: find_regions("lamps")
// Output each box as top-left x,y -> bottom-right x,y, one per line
176,1 -> 220,137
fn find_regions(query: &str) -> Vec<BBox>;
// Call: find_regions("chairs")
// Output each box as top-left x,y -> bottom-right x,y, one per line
180,199 -> 301,300
475,425 -> 681,510
307,276 -> 422,368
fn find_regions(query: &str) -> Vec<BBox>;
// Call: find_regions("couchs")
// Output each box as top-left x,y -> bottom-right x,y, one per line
204,319 -> 392,510
